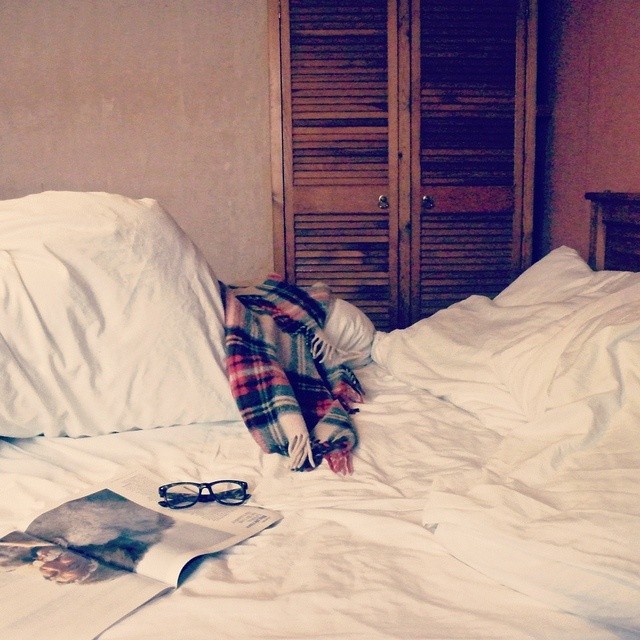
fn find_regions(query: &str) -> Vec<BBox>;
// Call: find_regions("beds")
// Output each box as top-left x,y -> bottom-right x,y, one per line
0,191 -> 640,640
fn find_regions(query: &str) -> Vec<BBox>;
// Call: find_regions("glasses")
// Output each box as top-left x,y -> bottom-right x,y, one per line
158,479 -> 252,509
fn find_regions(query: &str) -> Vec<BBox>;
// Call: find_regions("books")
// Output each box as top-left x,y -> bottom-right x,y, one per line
0,469 -> 283,640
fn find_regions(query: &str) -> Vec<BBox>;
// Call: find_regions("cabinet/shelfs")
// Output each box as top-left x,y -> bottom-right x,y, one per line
268,1 -> 539,330
